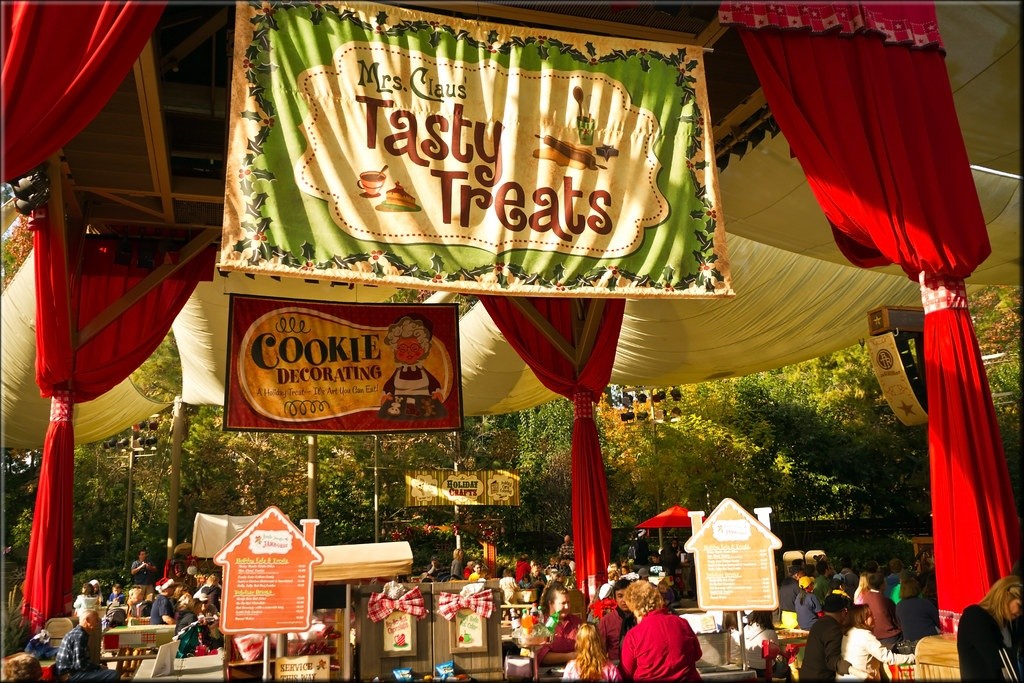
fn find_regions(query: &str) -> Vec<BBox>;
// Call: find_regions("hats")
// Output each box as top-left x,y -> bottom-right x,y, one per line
798,576 -> 815,589
155,578 -> 174,593
822,594 -> 848,612
530,559 -> 542,568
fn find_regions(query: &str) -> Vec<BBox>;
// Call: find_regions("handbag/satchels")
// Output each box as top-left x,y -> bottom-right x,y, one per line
234,634 -> 263,661
504,650 -> 534,681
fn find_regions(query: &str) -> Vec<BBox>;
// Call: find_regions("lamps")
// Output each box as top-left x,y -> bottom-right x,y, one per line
12,163 -> 51,217
115,238 -> 157,268
714,106 -> 781,173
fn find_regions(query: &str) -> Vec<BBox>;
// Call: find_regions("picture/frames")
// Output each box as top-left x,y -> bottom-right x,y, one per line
449,609 -> 488,653
376,612 -> 417,658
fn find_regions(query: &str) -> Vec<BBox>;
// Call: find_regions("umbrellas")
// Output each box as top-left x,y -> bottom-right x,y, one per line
635,505 -> 707,527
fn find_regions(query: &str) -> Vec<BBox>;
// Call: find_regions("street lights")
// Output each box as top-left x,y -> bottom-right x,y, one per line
102,413 -> 162,574
618,386 -> 682,553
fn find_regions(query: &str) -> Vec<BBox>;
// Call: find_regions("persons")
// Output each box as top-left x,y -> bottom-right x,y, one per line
532,581 -> 585,665
957,576 -> 1024,683
561,622 -> 623,682
586,528 -> 703,682
73,579 -> 125,617
427,548 -> 488,581
721,548 -> 941,683
131,550 -> 158,599
127,573 -> 222,637
519,535 -> 576,589
55,610 -> 120,683
499,568 -> 518,603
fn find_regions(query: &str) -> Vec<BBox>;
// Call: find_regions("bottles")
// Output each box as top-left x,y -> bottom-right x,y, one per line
510,608 -> 520,632
521,609 -> 532,634
530,603 -> 539,625
545,610 -> 560,633
538,606 -> 544,626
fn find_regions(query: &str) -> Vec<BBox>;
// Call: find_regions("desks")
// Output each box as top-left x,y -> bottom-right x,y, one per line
880,662 -> 916,683
772,628 -> 810,682
0,605 -> 225,683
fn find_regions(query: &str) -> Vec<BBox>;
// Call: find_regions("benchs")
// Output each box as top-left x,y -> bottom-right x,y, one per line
98,654 -> 156,680
500,604 -> 533,616
